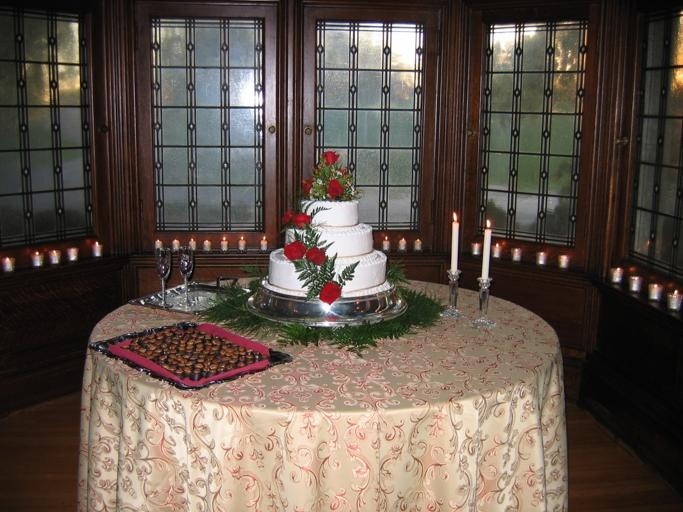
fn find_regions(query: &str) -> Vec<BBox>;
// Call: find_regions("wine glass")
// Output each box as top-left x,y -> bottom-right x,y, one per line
153,246 -> 173,309
178,245 -> 196,308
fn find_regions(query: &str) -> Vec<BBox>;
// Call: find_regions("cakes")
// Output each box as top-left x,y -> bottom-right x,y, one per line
268,170 -> 388,293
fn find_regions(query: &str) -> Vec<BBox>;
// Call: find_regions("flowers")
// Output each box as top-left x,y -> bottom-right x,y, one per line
283,149 -> 359,306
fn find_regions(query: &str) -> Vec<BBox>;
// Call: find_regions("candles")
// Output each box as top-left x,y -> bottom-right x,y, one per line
1,234 -> 102,272
151,235 -> 269,254
450,211 -> 493,279
